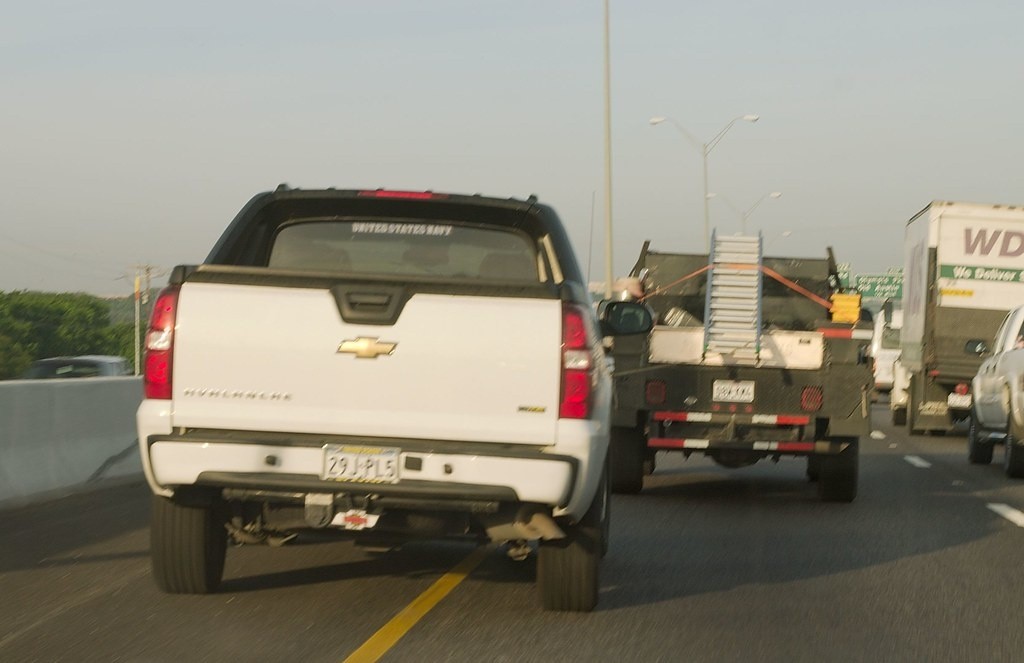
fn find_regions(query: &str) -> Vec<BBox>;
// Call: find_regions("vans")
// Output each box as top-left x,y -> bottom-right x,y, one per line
868,307 -> 906,393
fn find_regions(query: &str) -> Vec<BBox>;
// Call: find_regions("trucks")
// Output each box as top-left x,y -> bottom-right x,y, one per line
883,201 -> 1024,434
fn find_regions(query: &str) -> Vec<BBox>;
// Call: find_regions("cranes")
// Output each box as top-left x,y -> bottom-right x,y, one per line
114,261 -> 172,297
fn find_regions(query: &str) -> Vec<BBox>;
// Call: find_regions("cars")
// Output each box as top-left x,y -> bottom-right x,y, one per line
963,305 -> 1024,476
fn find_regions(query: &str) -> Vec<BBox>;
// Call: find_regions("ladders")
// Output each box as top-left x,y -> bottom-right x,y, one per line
700,226 -> 764,368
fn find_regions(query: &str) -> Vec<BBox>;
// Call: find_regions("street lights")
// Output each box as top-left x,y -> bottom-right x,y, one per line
705,189 -> 782,238
648,111 -> 759,255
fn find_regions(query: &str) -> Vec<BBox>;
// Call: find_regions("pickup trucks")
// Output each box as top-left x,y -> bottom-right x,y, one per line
134,183 -> 654,613
590,239 -> 877,502
